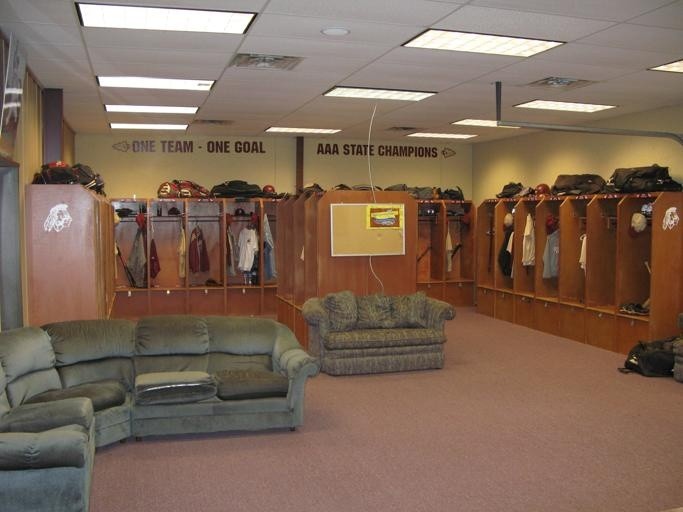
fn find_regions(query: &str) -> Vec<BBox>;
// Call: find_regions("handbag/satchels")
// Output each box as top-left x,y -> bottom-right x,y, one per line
354,184 -> 466,201
496,182 -> 534,199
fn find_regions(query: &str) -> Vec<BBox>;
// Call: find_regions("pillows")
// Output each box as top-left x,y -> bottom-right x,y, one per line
0,365 -> 289,472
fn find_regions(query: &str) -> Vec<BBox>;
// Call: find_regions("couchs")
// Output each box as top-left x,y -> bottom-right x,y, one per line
0,315 -> 320,511
301,289 -> 455,377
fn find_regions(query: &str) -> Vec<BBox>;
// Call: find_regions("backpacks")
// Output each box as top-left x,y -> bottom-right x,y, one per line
94,173 -> 105,192
552,172 -> 607,195
35,158 -> 78,185
71,162 -> 96,190
623,335 -> 679,377
602,167 -> 682,192
158,178 -> 264,199
299,183 -> 351,197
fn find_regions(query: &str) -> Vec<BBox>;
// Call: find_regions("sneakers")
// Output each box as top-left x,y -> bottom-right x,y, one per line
418,207 -> 467,218
205,278 -> 222,286
618,302 -> 650,316
167,206 -> 185,218
640,204 -> 653,214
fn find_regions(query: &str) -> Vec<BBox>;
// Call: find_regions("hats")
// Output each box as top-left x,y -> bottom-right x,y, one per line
250,213 -> 259,225
264,185 -> 275,194
535,183 -> 550,197
545,213 -> 555,234
135,214 -> 145,230
503,213 -> 513,232
226,213 -> 232,226
628,213 -> 647,238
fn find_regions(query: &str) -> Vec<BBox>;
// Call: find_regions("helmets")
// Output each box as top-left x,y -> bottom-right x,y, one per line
234,208 -> 245,215
